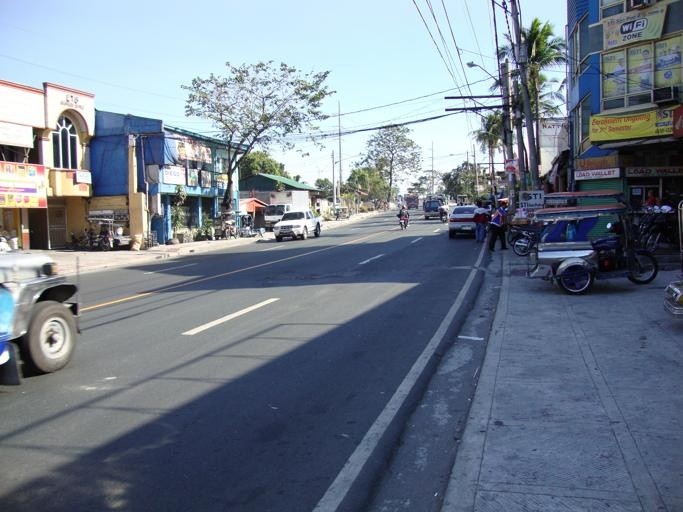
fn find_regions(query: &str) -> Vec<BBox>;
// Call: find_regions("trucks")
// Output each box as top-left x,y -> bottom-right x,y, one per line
264,190 -> 309,232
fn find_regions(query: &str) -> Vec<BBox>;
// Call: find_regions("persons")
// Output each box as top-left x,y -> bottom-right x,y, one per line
645,190 -> 657,206
399,204 -> 409,221
69,225 -> 108,250
488,199 -> 511,252
472,201 -> 487,243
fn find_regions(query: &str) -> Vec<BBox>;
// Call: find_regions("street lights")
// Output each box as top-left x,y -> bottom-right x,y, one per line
467,63 -> 516,215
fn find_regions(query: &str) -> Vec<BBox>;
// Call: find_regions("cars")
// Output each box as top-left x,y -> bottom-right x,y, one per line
0,249 -> 82,387
273,209 -> 323,242
405,194 -> 492,239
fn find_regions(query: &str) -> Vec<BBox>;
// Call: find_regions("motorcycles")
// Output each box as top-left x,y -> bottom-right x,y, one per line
635,205 -> 683,253
397,212 -> 409,230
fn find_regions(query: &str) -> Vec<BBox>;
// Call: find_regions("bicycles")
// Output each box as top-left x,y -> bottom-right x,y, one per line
507,226 -> 538,256
225,223 -> 239,240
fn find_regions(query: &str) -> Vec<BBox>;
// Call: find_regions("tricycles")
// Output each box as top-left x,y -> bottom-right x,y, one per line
525,189 -> 658,295
